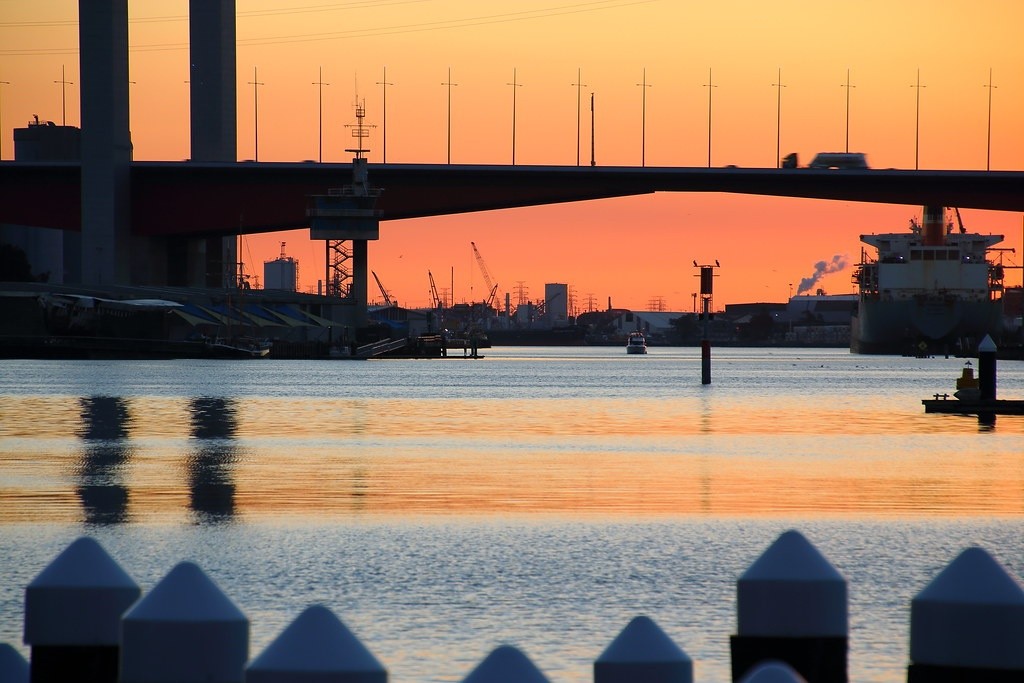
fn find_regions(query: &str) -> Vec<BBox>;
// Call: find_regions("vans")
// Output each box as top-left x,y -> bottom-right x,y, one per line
810,151 -> 868,170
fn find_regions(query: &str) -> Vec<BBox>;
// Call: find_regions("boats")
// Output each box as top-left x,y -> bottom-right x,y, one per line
848,204 -> 1005,356
626,332 -> 648,355
